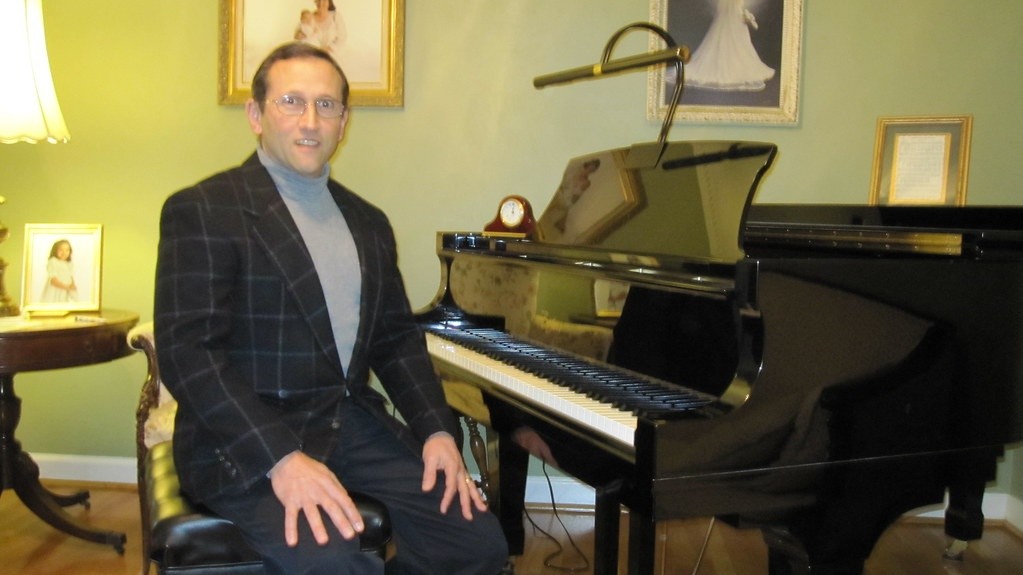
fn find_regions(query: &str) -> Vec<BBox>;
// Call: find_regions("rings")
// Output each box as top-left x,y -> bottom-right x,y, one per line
465,478 -> 472,482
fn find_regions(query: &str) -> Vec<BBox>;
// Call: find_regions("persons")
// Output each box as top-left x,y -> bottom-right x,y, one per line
154,43 -> 509,575
42,239 -> 80,301
294,0 -> 347,54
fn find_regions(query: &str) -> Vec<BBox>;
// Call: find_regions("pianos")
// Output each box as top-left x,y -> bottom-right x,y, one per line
415,20 -> 1023,574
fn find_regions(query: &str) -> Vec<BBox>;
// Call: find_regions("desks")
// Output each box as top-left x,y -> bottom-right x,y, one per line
1,311 -> 138,557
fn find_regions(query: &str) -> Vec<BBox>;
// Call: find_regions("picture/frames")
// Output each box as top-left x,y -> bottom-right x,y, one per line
22,223 -> 103,314
867,113 -> 975,206
650,0 -> 805,127
217,0 -> 408,110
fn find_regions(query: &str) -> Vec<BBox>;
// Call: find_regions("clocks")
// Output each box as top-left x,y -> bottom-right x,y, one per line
481,195 -> 543,241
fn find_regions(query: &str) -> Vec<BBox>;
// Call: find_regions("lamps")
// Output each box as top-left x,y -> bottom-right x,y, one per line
1,0 -> 67,318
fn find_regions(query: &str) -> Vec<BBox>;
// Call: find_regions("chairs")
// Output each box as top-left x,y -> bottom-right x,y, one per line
126,323 -> 395,575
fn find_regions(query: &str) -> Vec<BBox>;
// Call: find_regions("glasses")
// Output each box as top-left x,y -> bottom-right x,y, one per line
265,96 -> 345,118
